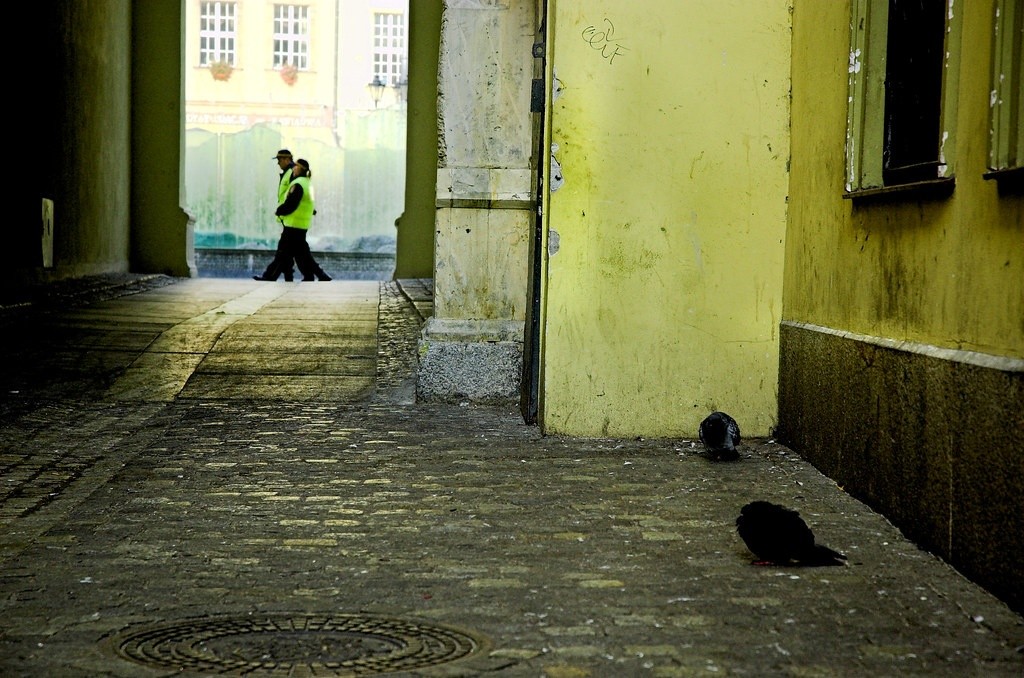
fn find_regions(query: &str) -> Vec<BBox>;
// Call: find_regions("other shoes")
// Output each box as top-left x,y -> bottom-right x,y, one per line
253,275 -> 267,280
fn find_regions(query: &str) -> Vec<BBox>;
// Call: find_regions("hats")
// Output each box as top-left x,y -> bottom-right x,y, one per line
272,150 -> 292,159
293,159 -> 308,171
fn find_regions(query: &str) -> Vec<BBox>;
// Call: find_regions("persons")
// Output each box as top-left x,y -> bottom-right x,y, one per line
255,150 -> 332,281
